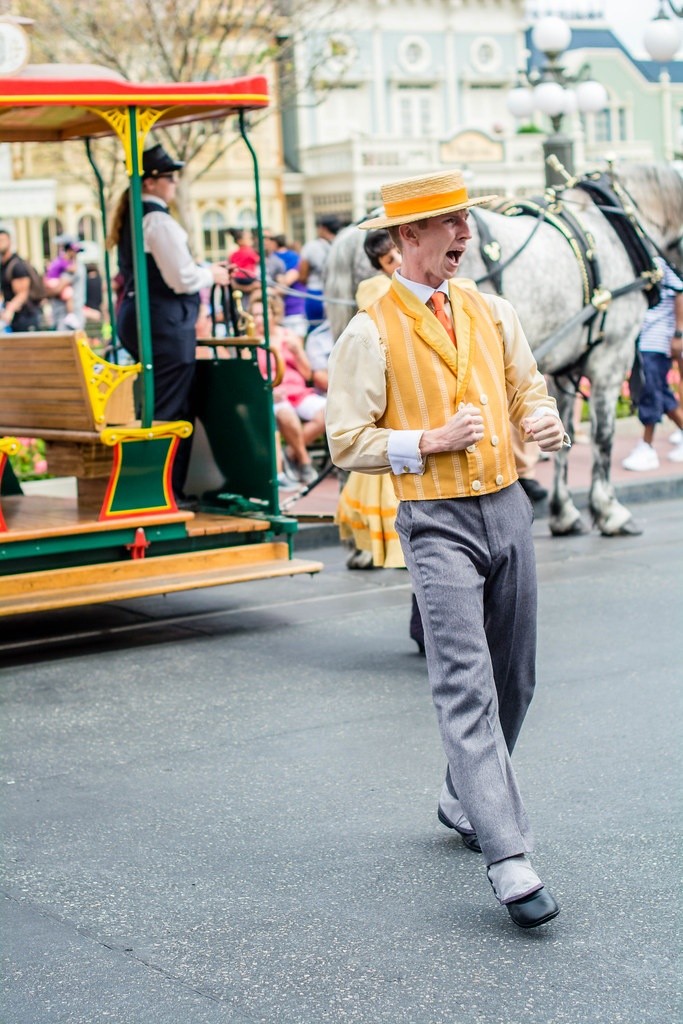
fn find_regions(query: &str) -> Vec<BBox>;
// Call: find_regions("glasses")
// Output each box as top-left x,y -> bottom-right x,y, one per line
153,171 -> 178,184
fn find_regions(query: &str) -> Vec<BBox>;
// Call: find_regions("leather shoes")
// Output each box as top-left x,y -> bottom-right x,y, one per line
437,805 -> 484,853
487,861 -> 557,926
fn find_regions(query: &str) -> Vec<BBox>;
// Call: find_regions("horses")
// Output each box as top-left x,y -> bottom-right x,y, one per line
319,154 -> 682,567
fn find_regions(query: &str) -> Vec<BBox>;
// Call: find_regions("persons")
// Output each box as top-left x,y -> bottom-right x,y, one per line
623,255 -> 682,473
323,167 -> 571,931
42,237 -> 85,333
1,229 -> 45,335
226,213 -> 341,343
103,142 -> 232,515
245,287 -> 335,491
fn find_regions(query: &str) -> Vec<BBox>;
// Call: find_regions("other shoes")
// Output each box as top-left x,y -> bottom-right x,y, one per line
622,445 -> 658,472
671,445 -> 683,463
173,490 -> 199,509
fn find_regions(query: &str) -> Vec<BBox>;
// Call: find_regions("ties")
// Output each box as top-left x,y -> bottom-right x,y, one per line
428,290 -> 456,344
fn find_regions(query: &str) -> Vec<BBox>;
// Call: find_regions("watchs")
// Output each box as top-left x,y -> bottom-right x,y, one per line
675,330 -> 682,338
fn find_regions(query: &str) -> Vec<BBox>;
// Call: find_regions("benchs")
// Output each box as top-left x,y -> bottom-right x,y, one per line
0,332 -> 194,524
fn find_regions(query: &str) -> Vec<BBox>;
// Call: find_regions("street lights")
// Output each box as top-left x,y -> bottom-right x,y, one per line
502,14 -> 610,183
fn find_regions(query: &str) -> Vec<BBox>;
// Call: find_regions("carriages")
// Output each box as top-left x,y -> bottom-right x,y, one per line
0,12 -> 682,618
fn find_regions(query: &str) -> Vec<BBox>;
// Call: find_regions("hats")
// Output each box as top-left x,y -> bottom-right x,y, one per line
356,168 -> 497,229
64,244 -> 82,253
124,142 -> 184,179
